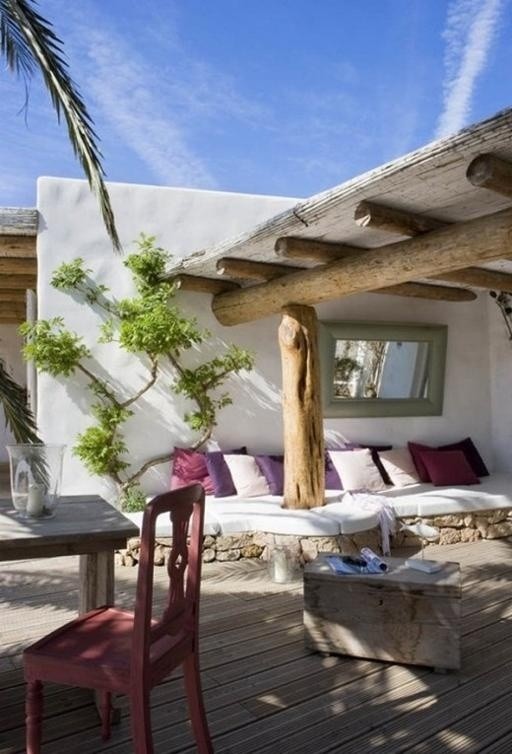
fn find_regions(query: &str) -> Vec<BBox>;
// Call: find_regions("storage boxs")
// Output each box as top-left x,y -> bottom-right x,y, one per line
303,552 -> 462,675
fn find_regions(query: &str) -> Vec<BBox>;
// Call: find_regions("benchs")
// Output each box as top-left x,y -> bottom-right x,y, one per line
114,472 -> 512,567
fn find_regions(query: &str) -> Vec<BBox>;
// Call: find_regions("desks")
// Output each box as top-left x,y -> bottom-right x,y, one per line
0,494 -> 140,725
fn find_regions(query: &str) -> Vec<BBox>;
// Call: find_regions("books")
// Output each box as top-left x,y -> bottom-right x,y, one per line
322,545 -> 389,576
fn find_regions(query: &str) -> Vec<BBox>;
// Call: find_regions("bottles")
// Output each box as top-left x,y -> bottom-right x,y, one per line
360,546 -> 390,572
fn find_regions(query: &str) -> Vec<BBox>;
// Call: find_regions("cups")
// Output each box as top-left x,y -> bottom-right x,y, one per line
5,443 -> 66,519
263,535 -> 298,584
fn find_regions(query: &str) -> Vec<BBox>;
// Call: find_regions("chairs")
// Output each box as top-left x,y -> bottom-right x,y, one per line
23,484 -> 213,754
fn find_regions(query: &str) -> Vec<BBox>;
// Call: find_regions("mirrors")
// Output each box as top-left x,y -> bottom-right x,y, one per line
318,320 -> 448,419
